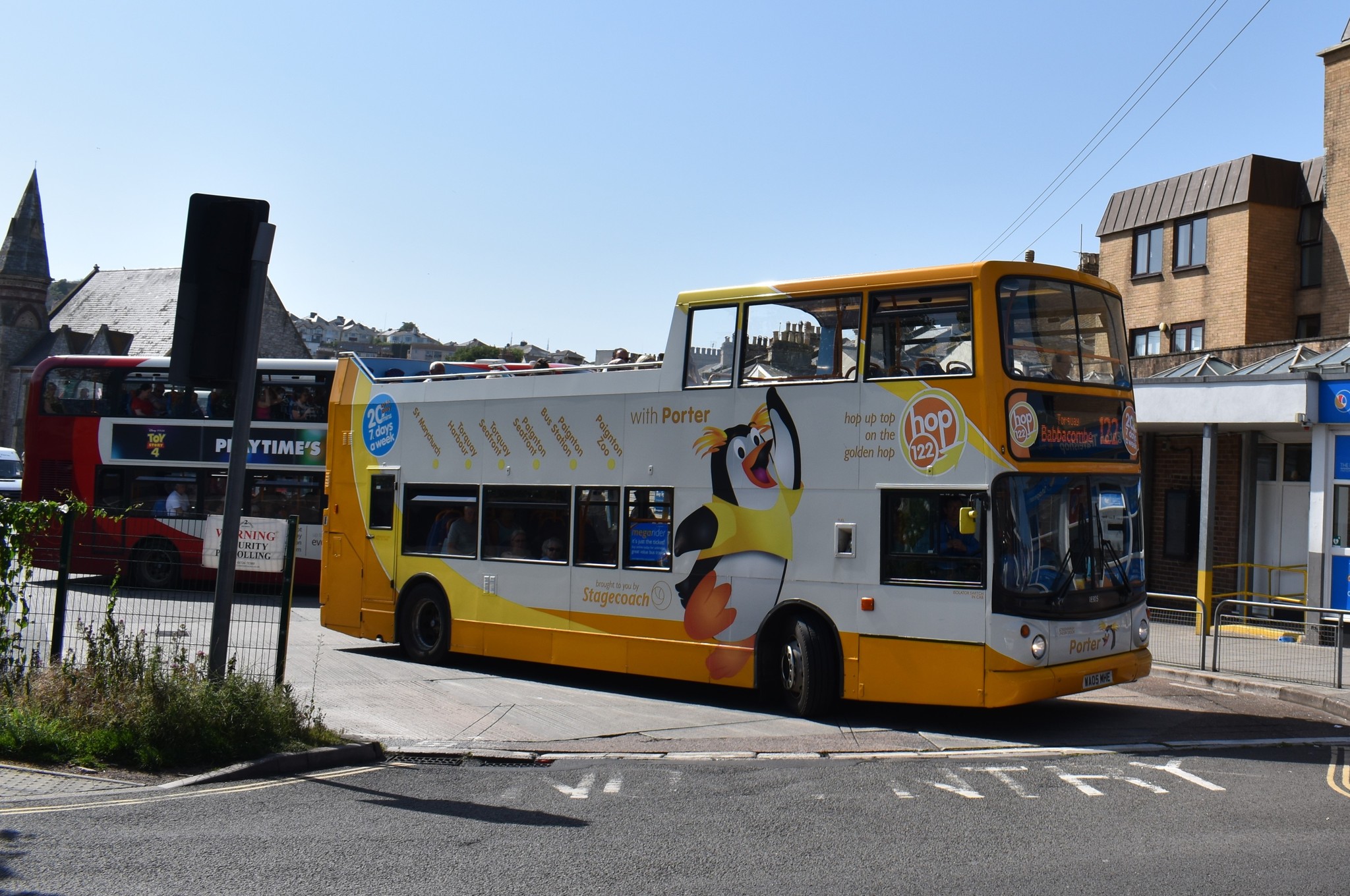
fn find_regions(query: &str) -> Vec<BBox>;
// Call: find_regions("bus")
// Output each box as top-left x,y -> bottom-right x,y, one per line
318,251 -> 1154,721
18,354 -> 598,594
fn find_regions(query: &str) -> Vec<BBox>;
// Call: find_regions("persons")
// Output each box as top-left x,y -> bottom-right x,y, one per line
1001,521 -> 1064,595
1043,354 -> 1074,381
422,349 -> 664,383
163,482 -> 323,523
427,484 -> 663,560
913,499 -> 981,582
45,381 -> 334,420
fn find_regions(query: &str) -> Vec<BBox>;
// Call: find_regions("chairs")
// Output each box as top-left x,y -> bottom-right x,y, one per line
841,358 -> 1047,378
425,509 -> 458,555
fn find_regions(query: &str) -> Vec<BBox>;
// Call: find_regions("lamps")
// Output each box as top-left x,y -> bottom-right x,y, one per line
1159,322 -> 1167,332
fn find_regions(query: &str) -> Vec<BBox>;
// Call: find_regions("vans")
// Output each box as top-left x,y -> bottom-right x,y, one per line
0,447 -> 24,502
